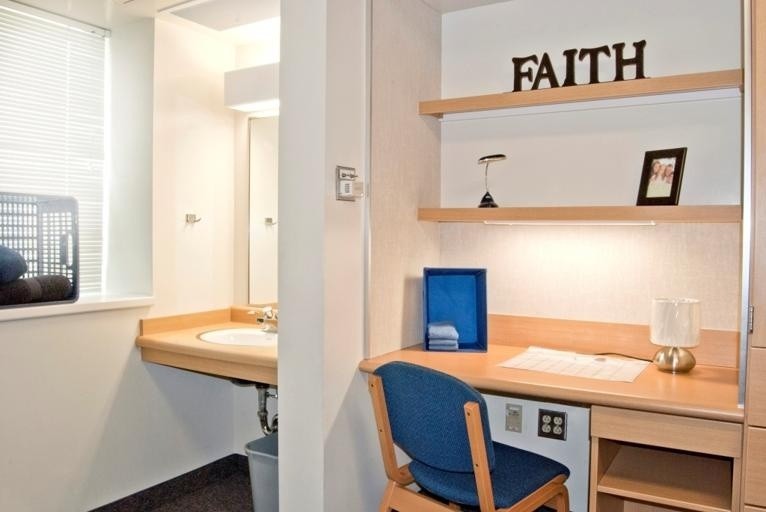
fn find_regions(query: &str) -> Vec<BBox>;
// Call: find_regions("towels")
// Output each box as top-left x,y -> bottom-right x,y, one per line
1,245 -> 28,284
0,275 -> 72,306
427,322 -> 459,352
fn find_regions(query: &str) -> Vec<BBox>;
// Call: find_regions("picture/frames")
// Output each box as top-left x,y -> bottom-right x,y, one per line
636,146 -> 686,206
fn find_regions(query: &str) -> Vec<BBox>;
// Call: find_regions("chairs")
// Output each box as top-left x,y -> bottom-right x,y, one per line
368,360 -> 570,512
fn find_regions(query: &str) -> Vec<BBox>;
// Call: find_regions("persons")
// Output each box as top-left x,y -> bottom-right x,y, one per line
649,161 -> 661,182
655,164 -> 665,183
662,164 -> 674,184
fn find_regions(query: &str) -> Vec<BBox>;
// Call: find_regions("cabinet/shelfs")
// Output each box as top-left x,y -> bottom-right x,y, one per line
417,67 -> 742,223
358,313 -> 746,512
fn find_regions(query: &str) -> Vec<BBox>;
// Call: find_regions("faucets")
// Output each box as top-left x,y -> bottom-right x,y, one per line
244,306 -> 278,324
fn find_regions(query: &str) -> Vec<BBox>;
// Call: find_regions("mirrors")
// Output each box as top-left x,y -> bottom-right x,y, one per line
249,115 -> 280,305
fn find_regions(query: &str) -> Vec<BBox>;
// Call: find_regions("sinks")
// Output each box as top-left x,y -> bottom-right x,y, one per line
196,325 -> 278,347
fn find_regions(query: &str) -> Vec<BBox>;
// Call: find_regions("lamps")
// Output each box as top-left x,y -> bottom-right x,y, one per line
649,298 -> 701,374
477,154 -> 506,208
